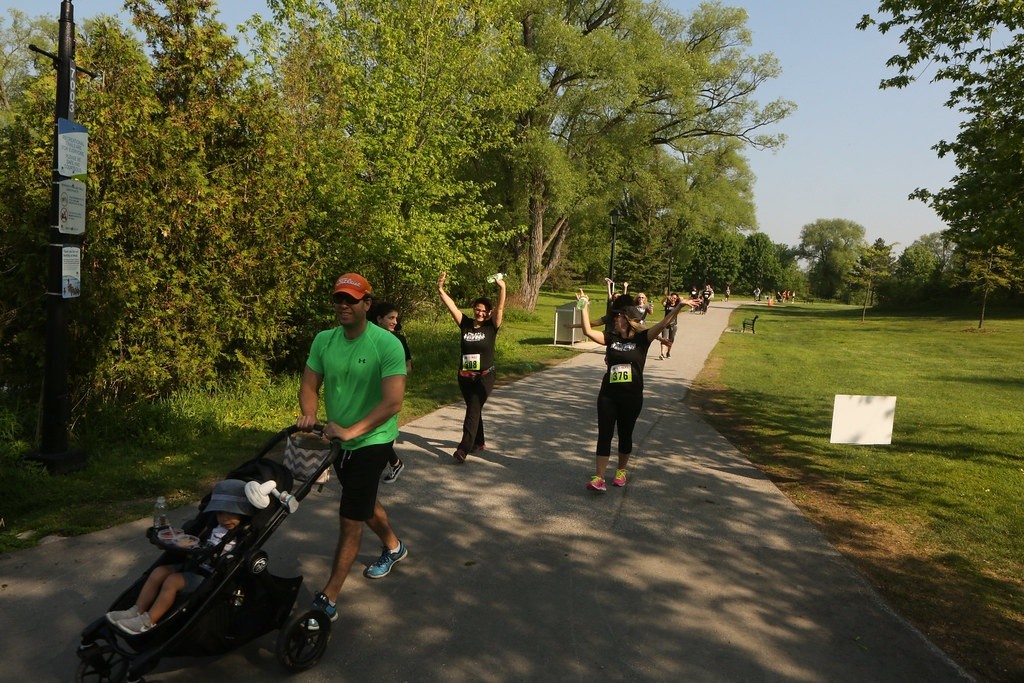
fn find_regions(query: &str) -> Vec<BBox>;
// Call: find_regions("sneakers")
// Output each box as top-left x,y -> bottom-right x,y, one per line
301,593 -> 338,630
117,612 -> 156,635
366,539 -> 408,578
383,458 -> 404,483
106,605 -> 141,624
612,469 -> 626,486
587,476 -> 606,492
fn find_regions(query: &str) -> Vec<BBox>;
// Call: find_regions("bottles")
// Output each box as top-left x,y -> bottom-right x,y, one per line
649,301 -> 653,310
576,295 -> 587,310
152,495 -> 167,528
486,272 -> 507,283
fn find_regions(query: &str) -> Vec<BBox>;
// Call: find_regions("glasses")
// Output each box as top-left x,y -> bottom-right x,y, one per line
333,295 -> 367,304
615,313 -> 625,318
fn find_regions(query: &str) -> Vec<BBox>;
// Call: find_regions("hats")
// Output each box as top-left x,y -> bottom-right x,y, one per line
333,274 -> 371,299
203,479 -> 254,517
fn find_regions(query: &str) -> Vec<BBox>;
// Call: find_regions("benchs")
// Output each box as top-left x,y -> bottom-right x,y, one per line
807,299 -> 814,304
743,315 -> 759,334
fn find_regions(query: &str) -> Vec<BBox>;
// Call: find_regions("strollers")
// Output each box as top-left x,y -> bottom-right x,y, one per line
76,422 -> 344,683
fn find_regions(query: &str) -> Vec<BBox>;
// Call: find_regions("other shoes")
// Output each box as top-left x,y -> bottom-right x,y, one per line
666,352 -> 671,358
454,448 -> 467,462
659,354 -> 664,360
469,444 -> 486,453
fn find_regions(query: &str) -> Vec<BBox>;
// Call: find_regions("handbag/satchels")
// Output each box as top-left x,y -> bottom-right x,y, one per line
283,428 -> 331,484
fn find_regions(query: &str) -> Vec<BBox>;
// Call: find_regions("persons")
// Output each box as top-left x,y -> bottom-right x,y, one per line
437,273 -> 506,463
297,273 -> 407,630
689,284 -> 714,312
776,289 -> 795,303
754,287 -> 760,301
724,284 -> 732,302
375,302 -> 411,483
563,281 -> 672,365
575,288 -> 703,494
106,479 -> 252,635
659,293 -> 682,360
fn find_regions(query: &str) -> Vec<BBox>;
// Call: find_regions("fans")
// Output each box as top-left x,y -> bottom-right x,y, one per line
244,479 -> 299,514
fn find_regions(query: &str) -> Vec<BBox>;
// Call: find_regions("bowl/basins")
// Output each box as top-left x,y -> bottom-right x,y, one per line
157,528 -> 184,545
171,535 -> 200,549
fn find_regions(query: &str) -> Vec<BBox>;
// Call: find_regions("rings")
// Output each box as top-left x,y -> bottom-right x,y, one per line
324,433 -> 328,435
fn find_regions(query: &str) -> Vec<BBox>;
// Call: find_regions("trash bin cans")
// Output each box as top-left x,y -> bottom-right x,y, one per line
769,299 -> 773,306
554,301 -> 587,346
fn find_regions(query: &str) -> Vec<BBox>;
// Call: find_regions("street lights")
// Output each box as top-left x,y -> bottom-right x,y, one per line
667,244 -> 675,295
606,208 -> 622,314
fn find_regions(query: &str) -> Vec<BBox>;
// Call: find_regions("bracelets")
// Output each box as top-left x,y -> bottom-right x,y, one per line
675,303 -> 684,310
